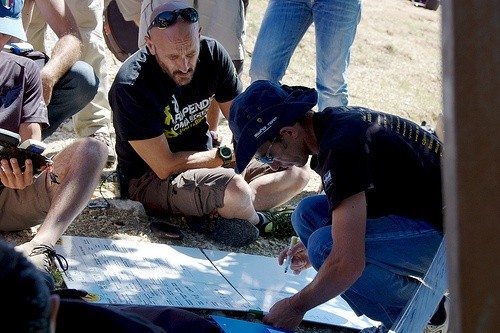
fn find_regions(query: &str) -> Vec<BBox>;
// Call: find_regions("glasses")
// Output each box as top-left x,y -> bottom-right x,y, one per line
146,7 -> 200,38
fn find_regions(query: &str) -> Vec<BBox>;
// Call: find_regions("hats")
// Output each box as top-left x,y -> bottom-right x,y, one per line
0,0 -> 29,41
228,79 -> 318,175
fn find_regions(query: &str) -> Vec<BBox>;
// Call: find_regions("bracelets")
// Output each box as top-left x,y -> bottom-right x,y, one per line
287,297 -> 306,316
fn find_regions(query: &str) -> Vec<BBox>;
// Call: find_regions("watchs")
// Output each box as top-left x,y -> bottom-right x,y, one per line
218,146 -> 235,167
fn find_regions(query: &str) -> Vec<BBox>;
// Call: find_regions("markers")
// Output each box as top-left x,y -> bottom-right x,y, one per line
284,236 -> 297,273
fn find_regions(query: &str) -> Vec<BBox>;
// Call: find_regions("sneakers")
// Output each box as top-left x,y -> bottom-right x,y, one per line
258,208 -> 298,237
197,216 -> 259,247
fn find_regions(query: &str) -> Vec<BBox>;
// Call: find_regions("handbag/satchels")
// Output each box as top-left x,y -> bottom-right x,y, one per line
101,0 -> 140,64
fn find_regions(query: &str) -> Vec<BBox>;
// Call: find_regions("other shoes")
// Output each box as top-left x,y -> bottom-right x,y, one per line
425,289 -> 451,333
360,324 -> 391,333
14,239 -> 68,292
88,131 -> 116,163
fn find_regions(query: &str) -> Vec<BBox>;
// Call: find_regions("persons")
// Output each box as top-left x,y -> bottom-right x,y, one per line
0,240 -> 58,333
229,80 -> 442,331
109,1 -> 309,246
103,2 -> 139,62
0,0 -> 107,297
24,0 -> 115,163
0,0 -> 99,146
250,1 -> 361,112
